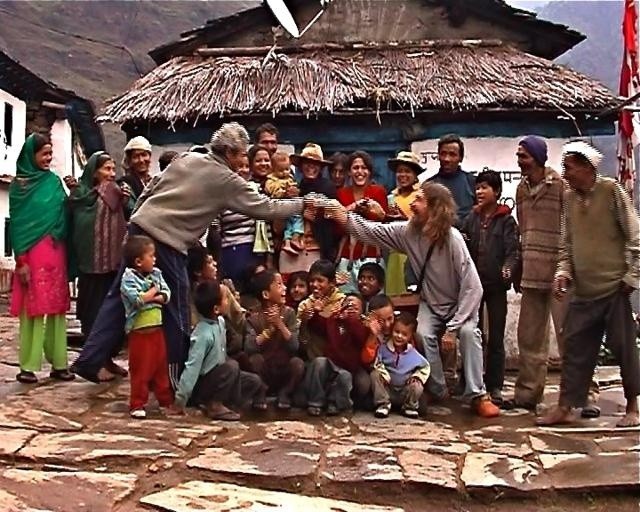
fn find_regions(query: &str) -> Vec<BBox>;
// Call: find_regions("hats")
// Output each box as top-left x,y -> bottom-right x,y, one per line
562,141 -> 604,170
387,150 -> 427,175
518,135 -> 547,166
290,142 -> 334,167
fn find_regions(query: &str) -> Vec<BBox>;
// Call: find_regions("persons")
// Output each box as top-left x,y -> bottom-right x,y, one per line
8,131 -> 77,382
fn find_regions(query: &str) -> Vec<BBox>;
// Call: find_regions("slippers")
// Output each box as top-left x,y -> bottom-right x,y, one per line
16,371 -> 37,383
211,407 -> 240,420
536,410 -> 576,427
251,399 -> 268,411
274,399 -> 291,409
616,410 -> 639,426
50,369 -> 75,381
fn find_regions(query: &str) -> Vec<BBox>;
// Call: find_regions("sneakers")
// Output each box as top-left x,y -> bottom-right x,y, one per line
327,402 -> 338,415
472,398 -> 499,416
97,368 -> 114,381
505,399 -> 535,409
581,403 -> 601,416
375,401 -> 392,416
159,407 -> 187,419
129,406 -> 146,418
106,359 -> 128,377
492,396 -> 503,408
400,409 -> 418,416
308,406 -> 320,416
